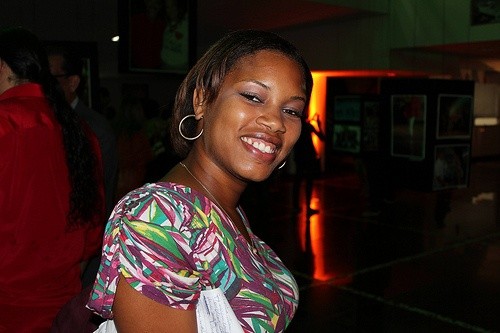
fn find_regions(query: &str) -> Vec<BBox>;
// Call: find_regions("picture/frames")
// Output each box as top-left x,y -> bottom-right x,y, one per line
436,94 -> 472,139
391,95 -> 426,161
431,144 -> 469,190
118,0 -> 199,75
68,41 -> 102,112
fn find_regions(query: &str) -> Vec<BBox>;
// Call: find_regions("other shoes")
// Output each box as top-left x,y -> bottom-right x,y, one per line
362,210 -> 381,217
384,198 -> 397,204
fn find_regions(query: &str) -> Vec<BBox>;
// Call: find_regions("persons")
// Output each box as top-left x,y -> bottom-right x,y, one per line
292,112 -> 325,215
39,41 -> 117,333
77,83 -> 185,206
85,30 -> 314,333
132,0 -> 188,68
0,27 -> 106,333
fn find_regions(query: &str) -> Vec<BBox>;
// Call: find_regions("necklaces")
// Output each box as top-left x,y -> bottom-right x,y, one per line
180,161 -> 257,253
